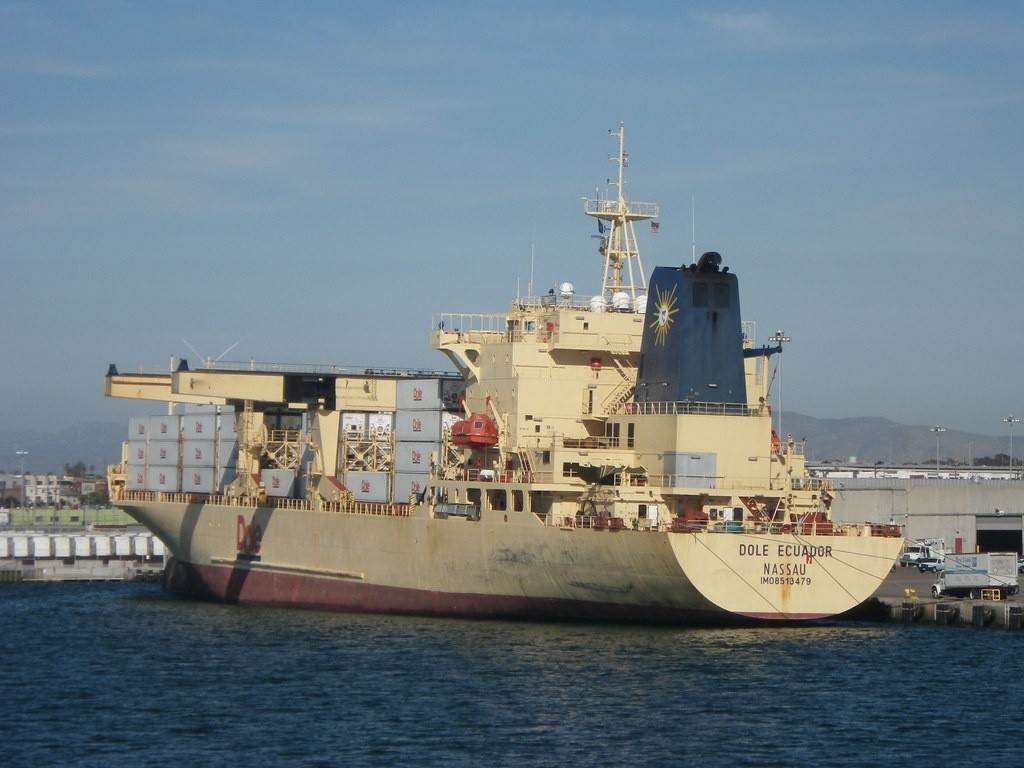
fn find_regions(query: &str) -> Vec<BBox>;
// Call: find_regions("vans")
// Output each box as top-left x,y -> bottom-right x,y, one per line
899,546 -> 934,566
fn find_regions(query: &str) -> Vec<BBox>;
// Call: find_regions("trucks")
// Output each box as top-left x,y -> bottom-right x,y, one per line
931,553 -> 1020,600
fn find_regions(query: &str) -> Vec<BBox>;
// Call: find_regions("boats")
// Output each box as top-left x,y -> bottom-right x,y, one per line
451,412 -> 498,446
105,119 -> 907,623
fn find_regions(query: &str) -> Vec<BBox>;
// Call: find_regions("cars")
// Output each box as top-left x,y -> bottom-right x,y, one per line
918,558 -> 946,572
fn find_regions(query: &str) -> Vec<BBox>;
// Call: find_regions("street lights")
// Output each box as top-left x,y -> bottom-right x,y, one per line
1003,414 -> 1020,481
769,330 -> 791,441
930,426 -> 946,479
16,449 -> 28,508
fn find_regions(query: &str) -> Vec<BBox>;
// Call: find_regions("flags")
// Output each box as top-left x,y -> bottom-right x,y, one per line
598,219 -> 606,234
650,220 -> 660,233
